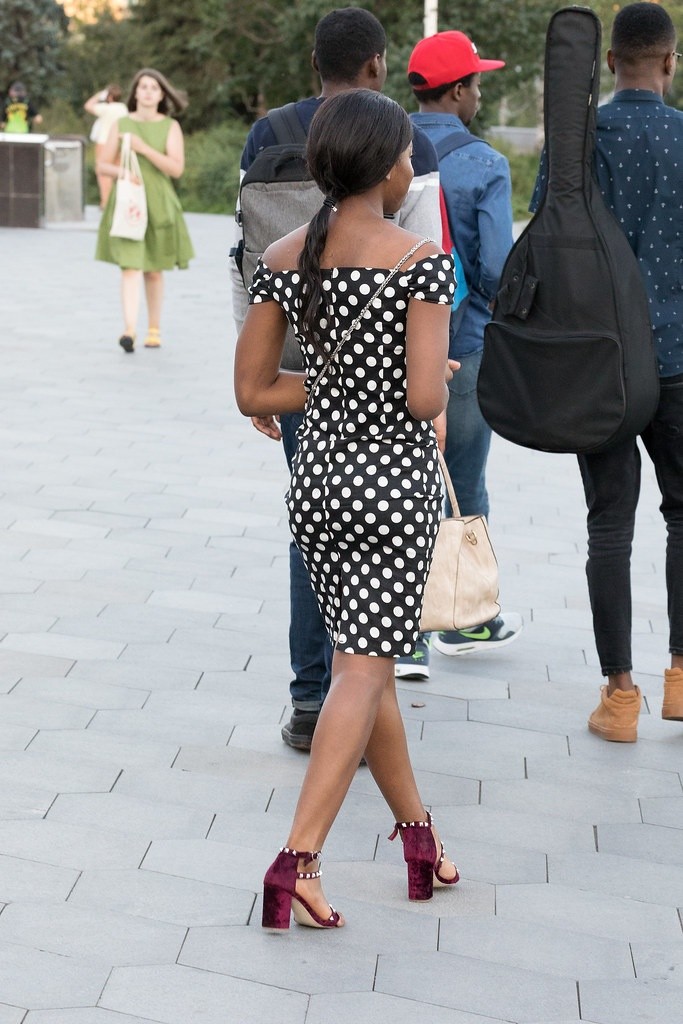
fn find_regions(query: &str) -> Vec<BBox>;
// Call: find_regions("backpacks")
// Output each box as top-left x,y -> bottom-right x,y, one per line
6,97 -> 29,134
230,101 -> 332,373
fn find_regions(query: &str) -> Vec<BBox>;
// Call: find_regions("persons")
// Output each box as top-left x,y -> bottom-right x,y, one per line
395,30 -> 522,680
97,68 -> 197,354
516,1 -> 683,743
236,81 -> 463,934
236,5 -> 460,761
0,80 -> 43,140
83,81 -> 130,210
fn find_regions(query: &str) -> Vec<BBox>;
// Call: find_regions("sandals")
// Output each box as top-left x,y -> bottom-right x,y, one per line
144,328 -> 161,348
120,332 -> 134,352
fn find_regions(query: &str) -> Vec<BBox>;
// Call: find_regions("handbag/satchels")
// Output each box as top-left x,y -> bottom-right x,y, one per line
109,133 -> 148,241
419,447 -> 501,632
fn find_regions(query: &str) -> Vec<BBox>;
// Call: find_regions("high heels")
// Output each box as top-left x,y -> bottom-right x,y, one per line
388,810 -> 460,903
261,847 -> 345,932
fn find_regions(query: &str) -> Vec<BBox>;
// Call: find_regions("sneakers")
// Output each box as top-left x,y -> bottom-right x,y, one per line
661,666 -> 682,720
431,612 -> 523,656
359,758 -> 367,766
394,633 -> 431,678
281,712 -> 319,750
588,684 -> 641,743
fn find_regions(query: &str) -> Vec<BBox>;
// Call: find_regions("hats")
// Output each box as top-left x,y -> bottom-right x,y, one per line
407,30 -> 505,91
